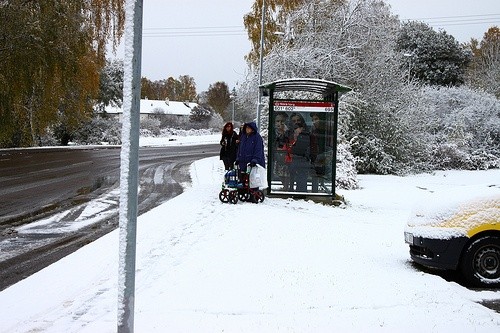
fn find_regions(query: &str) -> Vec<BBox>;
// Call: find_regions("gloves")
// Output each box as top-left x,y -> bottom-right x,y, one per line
248,162 -> 256,168
235,160 -> 239,165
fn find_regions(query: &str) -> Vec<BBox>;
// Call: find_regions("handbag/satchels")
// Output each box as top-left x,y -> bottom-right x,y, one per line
247,163 -> 269,190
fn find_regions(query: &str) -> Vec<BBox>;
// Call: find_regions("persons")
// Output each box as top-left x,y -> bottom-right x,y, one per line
219,122 -> 238,172
274,113 -> 327,176
235,122 -> 265,203
274,123 -> 325,192
236,123 -> 244,169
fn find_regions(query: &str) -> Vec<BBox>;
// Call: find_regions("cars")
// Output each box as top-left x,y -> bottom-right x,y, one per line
404,187 -> 500,288
